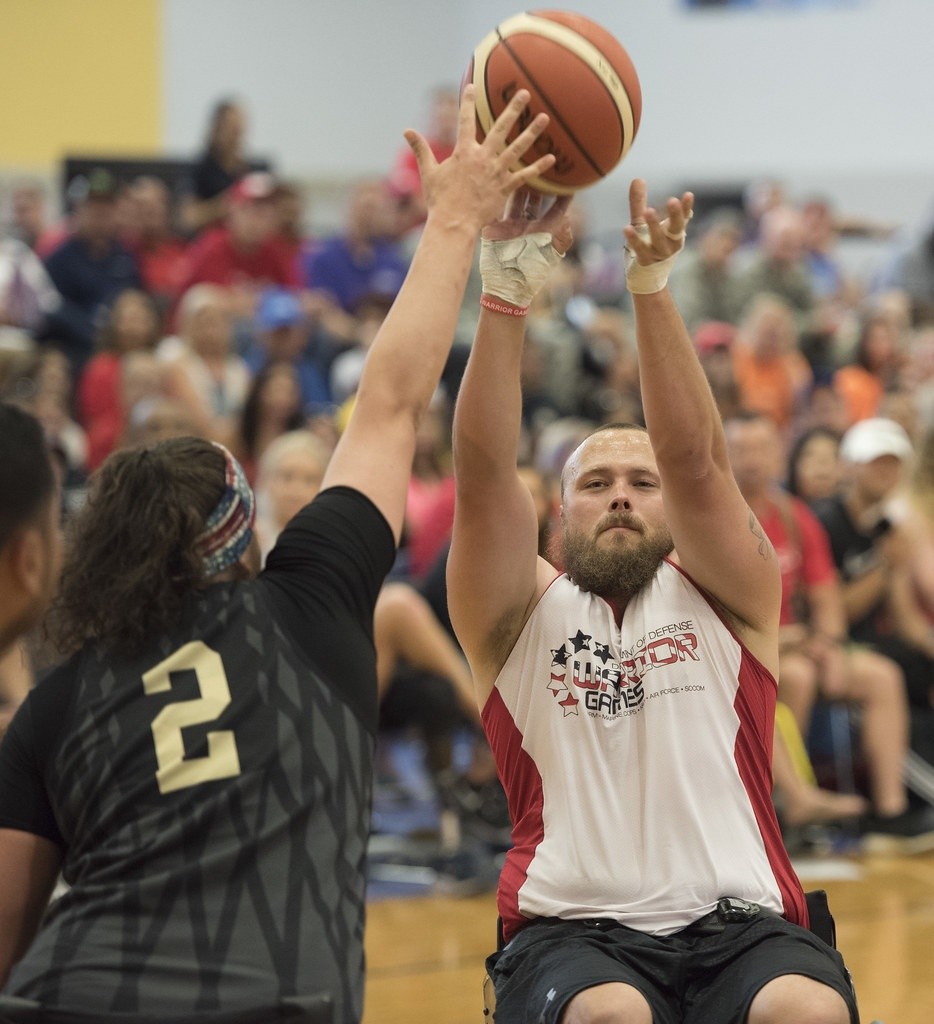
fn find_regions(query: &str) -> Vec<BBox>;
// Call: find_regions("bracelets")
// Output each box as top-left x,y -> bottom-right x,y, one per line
479,292 -> 527,319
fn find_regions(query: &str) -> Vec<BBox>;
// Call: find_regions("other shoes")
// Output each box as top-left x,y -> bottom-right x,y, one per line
859,805 -> 931,861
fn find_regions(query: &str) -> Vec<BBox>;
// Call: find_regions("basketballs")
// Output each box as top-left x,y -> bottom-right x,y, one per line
458,8 -> 644,197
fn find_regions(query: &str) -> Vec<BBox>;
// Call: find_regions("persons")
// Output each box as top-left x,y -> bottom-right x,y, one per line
0,401 -> 62,655
0,104 -> 934,892
0,86 -> 554,1023
442,181 -> 858,1024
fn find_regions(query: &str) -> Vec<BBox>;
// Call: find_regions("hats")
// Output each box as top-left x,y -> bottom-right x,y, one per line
841,416 -> 917,462
